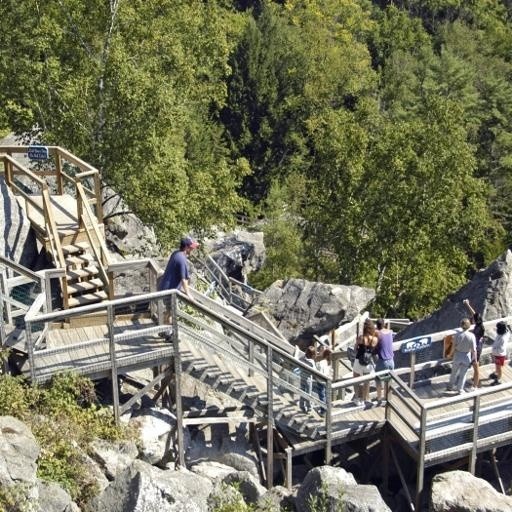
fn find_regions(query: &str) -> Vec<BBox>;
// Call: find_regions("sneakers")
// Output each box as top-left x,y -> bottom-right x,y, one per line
489,372 -> 499,378
448,378 -> 477,394
492,379 -> 500,385
353,397 -> 365,405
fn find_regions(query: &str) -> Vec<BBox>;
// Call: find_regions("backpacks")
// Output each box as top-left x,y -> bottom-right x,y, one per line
359,335 -> 372,366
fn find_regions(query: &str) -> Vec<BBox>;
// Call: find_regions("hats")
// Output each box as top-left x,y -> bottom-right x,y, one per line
181,236 -> 198,248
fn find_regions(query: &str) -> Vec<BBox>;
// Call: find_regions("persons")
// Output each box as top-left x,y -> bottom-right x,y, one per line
315,348 -> 333,416
158,234 -> 195,343
490,321 -> 509,385
299,346 -> 316,415
463,299 -> 484,392
352,318 -> 378,406
448,318 -> 477,395
375,318 -> 394,406
